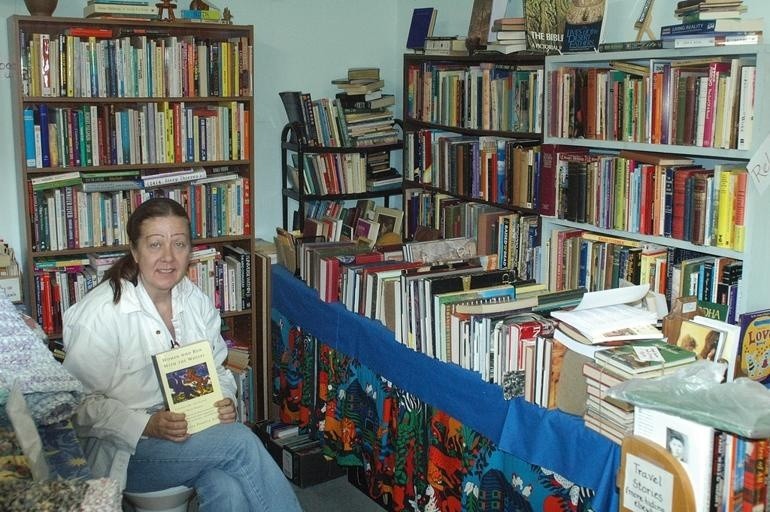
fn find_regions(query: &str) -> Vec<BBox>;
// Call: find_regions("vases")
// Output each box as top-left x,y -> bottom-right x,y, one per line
25,1 -> 60,15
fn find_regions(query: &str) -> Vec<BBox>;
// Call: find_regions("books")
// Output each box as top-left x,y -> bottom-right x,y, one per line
597,37 -> 664,54
485,16 -> 529,56
408,59 -> 768,509
17,28 -> 260,443
81,1 -> 163,23
181,10 -> 223,20
404,7 -> 438,52
273,64 -> 409,348
424,39 -> 468,52
659,0 -> 762,51
176,18 -> 221,24
425,50 -> 471,57
424,36 -> 458,41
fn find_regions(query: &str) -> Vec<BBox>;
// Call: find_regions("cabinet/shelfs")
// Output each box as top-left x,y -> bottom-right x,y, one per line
401,50 -> 555,284
279,118 -> 409,234
544,41 -> 769,331
5,14 -> 259,455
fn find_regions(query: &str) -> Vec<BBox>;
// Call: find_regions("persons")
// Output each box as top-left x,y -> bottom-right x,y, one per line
59,195 -> 309,512
